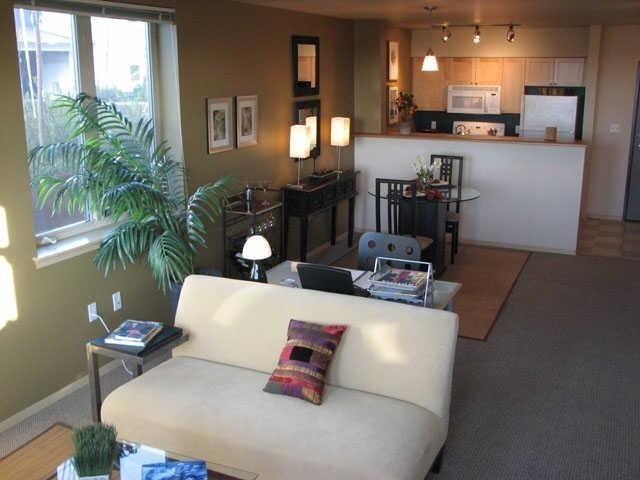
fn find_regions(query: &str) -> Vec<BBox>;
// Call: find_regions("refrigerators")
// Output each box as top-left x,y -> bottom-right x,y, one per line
519,95 -> 577,140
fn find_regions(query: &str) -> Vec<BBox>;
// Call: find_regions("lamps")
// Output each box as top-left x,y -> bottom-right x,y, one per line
330,117 -> 350,173
420,47 -> 441,72
240,234 -> 273,282
505,25 -> 518,44
471,26 -> 482,46
305,116 -> 317,145
289,125 -> 310,189
439,26 -> 453,48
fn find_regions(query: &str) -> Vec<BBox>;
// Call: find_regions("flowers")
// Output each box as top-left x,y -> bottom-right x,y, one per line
408,150 -> 442,186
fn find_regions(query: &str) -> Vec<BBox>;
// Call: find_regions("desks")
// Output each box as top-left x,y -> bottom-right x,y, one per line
266,261 -> 463,313
279,170 -> 361,262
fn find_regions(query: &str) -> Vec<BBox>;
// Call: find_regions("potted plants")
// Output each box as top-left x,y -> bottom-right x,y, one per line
69,421 -> 119,480
23,91 -> 256,323
393,90 -> 420,137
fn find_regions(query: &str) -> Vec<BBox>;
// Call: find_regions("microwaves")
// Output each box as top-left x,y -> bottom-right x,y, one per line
448,84 -> 501,115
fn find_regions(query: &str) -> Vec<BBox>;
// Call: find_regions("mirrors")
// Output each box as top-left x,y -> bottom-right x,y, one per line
290,35 -> 319,95
295,99 -> 322,161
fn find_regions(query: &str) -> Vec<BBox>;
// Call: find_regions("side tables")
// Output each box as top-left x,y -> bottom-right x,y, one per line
85,325 -> 190,423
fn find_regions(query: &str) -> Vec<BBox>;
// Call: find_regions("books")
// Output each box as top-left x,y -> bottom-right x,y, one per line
105,320 -> 164,350
116,318 -> 158,341
377,269 -> 427,286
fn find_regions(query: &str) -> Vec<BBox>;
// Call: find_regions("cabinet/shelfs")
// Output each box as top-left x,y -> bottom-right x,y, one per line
410,56 -> 585,113
222,192 -> 284,283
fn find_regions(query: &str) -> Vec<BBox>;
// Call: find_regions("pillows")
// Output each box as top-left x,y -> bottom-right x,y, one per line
262,318 -> 352,406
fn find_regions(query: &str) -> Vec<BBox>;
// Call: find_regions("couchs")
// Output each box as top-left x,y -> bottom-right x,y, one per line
100,274 -> 460,480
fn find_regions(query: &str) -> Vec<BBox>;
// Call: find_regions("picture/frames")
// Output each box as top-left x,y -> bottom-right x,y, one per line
385,85 -> 400,126
387,40 -> 400,82
235,94 -> 259,150
206,95 -> 236,154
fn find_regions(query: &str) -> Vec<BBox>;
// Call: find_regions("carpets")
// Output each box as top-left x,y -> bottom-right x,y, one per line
1,420 -> 80,480
325,242 -> 533,343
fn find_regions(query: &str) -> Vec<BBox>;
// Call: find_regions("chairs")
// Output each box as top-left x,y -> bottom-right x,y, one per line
375,178 -> 434,252
356,231 -> 421,271
429,154 -> 463,264
